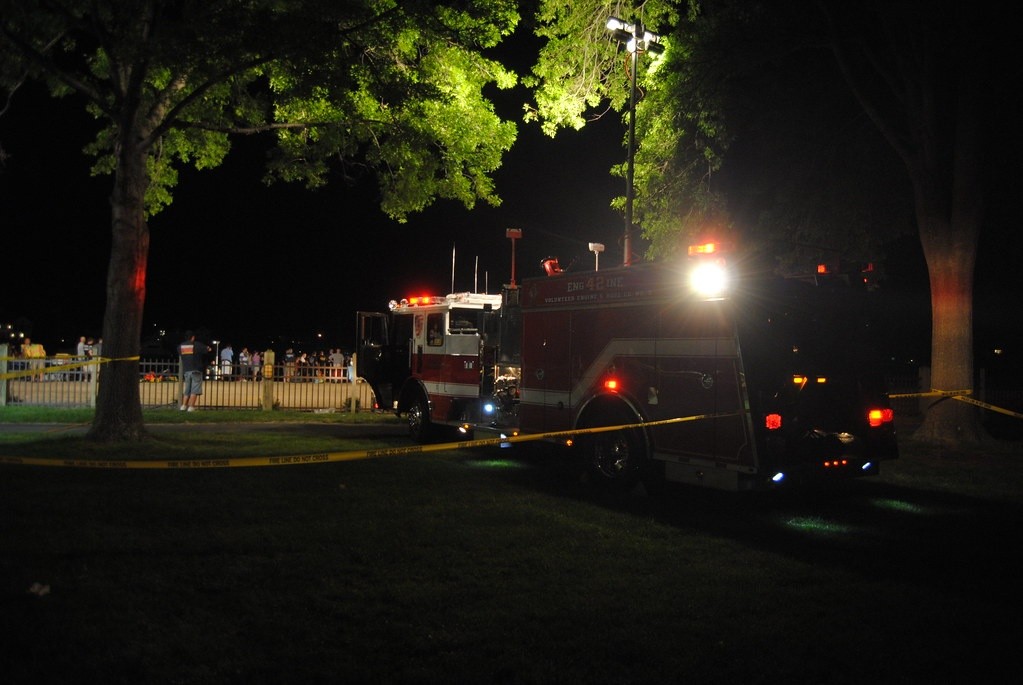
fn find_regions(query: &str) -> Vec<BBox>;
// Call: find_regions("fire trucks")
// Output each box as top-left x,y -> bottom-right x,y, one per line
355,245 -> 900,517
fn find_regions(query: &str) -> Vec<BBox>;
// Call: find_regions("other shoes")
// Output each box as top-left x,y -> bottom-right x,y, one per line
180,405 -> 188,411
186,407 -> 200,412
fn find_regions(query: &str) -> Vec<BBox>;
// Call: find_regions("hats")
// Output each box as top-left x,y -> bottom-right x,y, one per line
87,337 -> 93,341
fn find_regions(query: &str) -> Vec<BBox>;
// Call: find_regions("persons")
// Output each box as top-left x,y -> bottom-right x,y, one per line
428,325 -> 441,346
75,336 -> 103,378
177,330 -> 212,411
220,340 -> 356,383
19,338 -> 46,382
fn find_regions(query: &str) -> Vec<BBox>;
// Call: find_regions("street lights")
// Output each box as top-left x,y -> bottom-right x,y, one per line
607,15 -> 665,266
